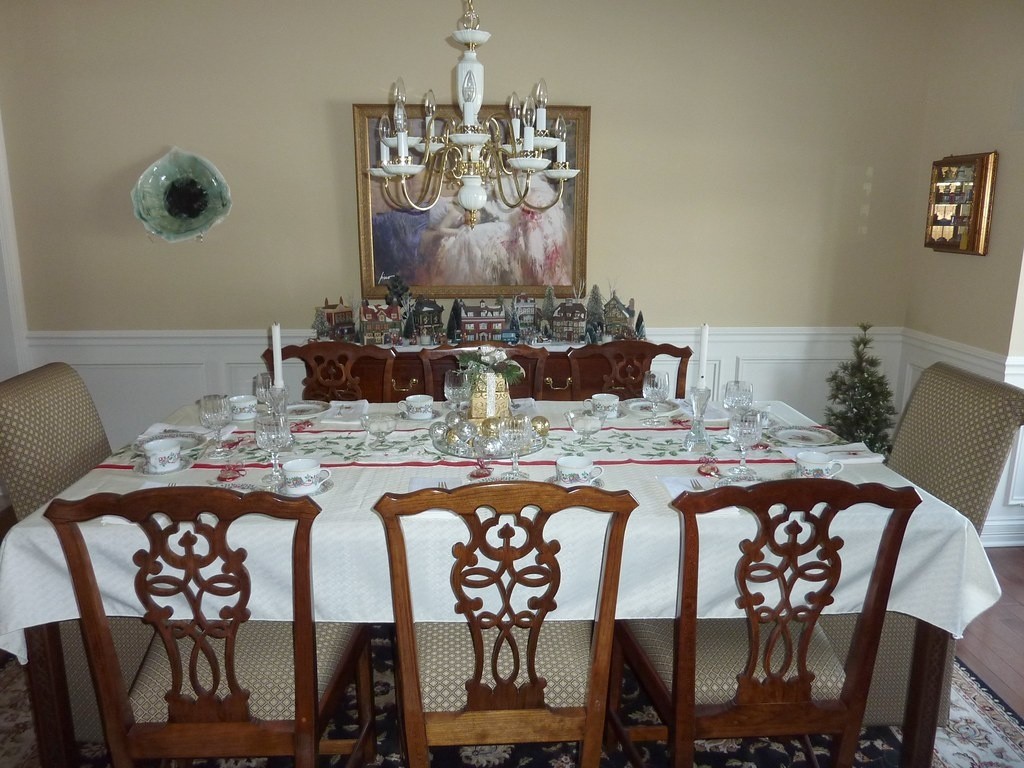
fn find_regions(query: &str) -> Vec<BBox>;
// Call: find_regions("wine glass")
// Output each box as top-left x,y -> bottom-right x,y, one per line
255,415 -> 292,486
727,413 -> 762,479
200,395 -> 234,460
256,373 -> 275,414
562,409 -> 607,447
445,369 -> 471,413
722,381 -> 753,441
642,371 -> 669,428
498,416 -> 533,480
360,414 -> 396,451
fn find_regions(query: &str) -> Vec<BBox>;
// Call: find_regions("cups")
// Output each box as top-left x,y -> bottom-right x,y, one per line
795,451 -> 841,480
282,459 -> 331,496
555,457 -> 603,486
584,393 -> 620,420
230,395 -> 256,422
397,394 -> 434,419
143,440 -> 181,473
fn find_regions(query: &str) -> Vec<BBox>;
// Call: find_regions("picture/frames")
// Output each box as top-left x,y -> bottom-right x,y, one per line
352,103 -> 593,301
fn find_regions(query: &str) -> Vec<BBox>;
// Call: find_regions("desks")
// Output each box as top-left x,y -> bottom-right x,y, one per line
0,400 -> 1003,768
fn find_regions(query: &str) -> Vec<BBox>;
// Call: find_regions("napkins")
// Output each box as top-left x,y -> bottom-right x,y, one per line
780,442 -> 884,466
322,400 -> 368,425
510,397 -> 538,419
137,423 -> 236,442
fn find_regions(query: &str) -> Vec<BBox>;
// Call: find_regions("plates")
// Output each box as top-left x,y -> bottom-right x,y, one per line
129,368 -> 842,498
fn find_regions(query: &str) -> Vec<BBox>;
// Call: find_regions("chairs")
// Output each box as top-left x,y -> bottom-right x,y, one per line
0,344 -> 1024,768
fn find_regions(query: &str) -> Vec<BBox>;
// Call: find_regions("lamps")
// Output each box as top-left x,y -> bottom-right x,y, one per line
359,0 -> 581,228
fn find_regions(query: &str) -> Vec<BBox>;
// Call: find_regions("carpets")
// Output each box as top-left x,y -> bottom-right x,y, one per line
0,624 -> 1024,768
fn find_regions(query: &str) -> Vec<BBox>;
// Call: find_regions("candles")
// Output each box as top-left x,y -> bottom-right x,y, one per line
272,321 -> 284,388
697,322 -> 708,390
